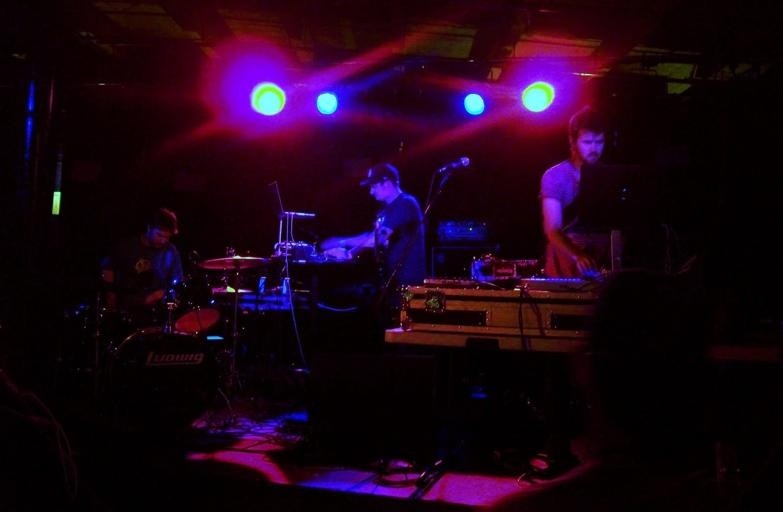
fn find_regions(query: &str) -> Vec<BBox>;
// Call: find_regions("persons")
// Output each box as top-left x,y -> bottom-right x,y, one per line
93,207 -> 188,334
318,161 -> 428,291
538,104 -> 626,280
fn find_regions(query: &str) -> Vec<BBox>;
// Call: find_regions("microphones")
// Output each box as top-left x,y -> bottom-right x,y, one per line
436,156 -> 471,173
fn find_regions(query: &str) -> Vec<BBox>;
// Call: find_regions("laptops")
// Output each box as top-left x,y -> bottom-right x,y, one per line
265,180 -> 317,218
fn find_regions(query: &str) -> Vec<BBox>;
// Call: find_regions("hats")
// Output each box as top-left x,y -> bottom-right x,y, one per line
360,163 -> 399,186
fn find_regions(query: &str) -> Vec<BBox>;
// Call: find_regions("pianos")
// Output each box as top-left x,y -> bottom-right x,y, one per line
209,286 -> 312,315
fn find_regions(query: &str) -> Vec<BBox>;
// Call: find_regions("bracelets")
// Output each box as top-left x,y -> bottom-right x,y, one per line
339,239 -> 347,248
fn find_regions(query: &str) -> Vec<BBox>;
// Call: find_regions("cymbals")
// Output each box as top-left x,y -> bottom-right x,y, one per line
197,255 -> 271,270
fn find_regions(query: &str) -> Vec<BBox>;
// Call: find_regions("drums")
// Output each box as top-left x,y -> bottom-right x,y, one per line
164,268 -> 229,337
104,326 -> 221,431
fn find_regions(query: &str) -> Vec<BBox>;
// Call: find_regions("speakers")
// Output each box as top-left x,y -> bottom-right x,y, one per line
431,245 -> 498,277
